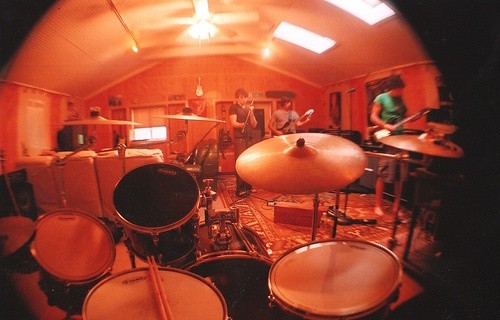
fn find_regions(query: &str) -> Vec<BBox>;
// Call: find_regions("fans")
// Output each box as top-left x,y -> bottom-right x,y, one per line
141,0 -> 260,39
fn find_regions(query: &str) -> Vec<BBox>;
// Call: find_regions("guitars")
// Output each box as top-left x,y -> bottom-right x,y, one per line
270,109 -> 314,136
372,108 -> 431,141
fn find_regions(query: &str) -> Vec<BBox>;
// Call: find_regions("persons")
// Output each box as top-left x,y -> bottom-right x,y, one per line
268,96 -> 311,136
370,77 -> 430,215
228,89 -> 257,198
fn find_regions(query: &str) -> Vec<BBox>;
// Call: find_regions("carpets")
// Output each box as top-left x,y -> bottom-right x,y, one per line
217,175 -> 422,261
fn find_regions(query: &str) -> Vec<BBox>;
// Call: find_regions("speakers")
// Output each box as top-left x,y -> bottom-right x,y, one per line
360,157 -> 380,189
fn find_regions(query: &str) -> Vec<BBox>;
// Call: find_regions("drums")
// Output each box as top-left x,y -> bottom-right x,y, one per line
194,141 -> 219,177
112,163 -> 201,264
168,209 -> 275,320
152,107 -> 227,126
27,207 -> 117,312
47,106 -> 142,130
267,239 -> 403,320
82,267 -> 229,319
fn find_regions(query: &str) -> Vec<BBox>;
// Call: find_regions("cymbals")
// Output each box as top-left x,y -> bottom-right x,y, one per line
377,134 -> 463,158
236,134 -> 369,195
0,217 -> 37,257
